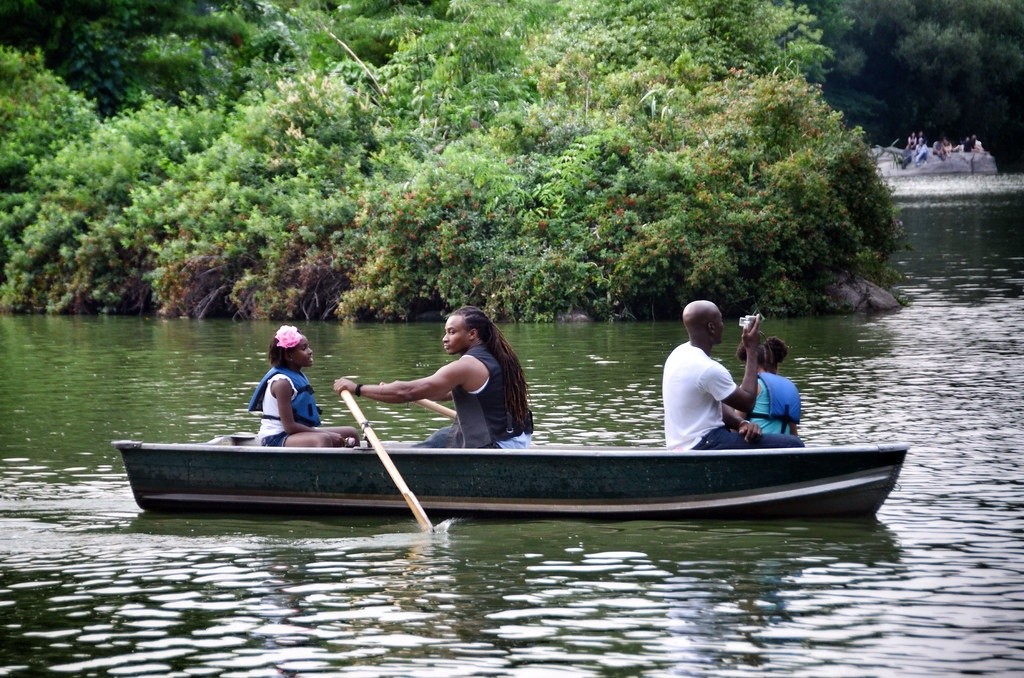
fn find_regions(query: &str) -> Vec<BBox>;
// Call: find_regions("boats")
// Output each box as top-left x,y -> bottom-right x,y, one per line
112,436 -> 912,522
870,146 -> 998,179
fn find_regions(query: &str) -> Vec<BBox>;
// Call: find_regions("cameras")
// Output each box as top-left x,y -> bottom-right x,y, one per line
739,315 -> 756,328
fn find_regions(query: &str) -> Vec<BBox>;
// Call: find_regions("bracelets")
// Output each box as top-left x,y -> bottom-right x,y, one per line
739,420 -> 750,426
355,384 -> 363,397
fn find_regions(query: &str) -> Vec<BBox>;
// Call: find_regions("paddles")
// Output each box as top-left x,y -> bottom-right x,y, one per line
340,388 -> 435,534
379,382 -> 457,419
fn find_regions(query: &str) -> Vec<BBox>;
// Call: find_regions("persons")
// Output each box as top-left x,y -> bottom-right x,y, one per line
662,301 -> 805,449
334,305 -> 534,449
248,322 -> 359,448
906,131 -> 987,167
731,336 -> 801,435
